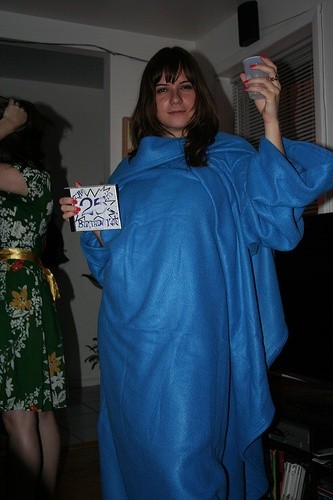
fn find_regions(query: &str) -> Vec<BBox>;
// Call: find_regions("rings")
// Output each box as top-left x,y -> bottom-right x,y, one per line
269,74 -> 279,81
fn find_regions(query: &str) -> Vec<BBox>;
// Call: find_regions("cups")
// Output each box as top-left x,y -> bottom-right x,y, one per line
242,56 -> 269,99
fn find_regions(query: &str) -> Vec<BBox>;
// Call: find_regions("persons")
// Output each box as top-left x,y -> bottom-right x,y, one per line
0,96 -> 68,500
59,47 -> 333,500
